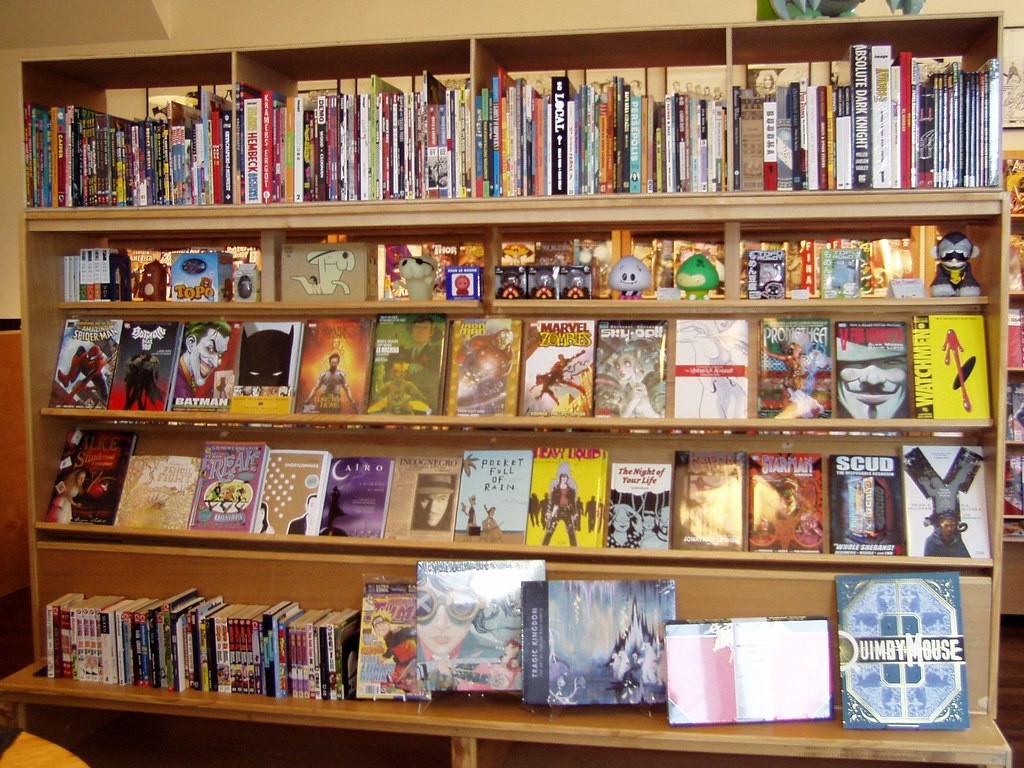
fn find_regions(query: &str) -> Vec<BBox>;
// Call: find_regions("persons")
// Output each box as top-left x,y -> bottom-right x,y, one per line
543,473 -> 581,548
411,474 -> 455,530
925,514 -> 970,558
45,469 -> 87,523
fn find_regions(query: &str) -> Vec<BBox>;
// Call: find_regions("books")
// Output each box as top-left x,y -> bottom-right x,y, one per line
26,45 -> 1002,208
49,314 -> 990,419
57,245 -> 258,301
359,561 -> 969,733
377,239 -> 484,302
500,237 -> 723,301
740,224 -> 956,300
42,590 -> 358,702
1002,161 -> 1024,543
44,427 -> 991,558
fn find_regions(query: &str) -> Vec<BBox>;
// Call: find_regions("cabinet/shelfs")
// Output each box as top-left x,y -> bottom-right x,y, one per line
1000,149 -> 1024,629
0,8 -> 1013,768
